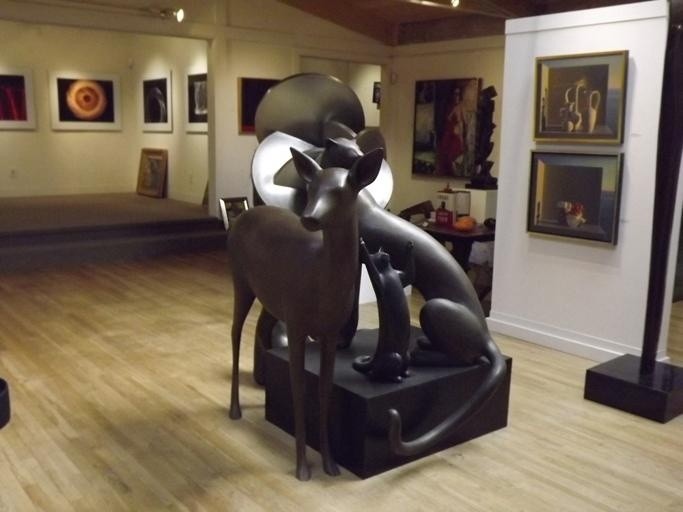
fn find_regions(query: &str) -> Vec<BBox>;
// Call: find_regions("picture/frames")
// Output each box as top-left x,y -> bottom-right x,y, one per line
139,70 -> 172,133
184,71 -> 208,134
136,148 -> 168,198
218,196 -> 249,231
0,67 -> 36,132
372,81 -> 382,104
532,50 -> 629,142
49,71 -> 123,133
525,147 -> 625,246
411,78 -> 481,179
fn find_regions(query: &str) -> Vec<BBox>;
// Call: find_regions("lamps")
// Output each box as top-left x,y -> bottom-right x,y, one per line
162,7 -> 184,23
238,75 -> 281,136
449,1 -> 460,9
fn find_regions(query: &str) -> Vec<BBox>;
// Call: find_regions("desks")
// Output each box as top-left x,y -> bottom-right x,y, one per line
417,221 -> 494,267
